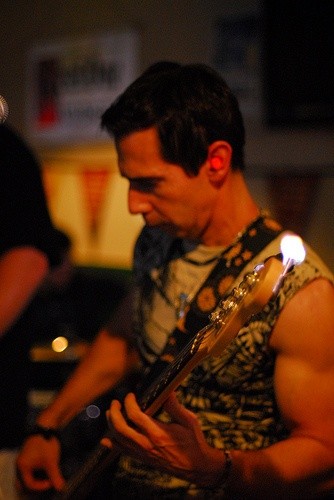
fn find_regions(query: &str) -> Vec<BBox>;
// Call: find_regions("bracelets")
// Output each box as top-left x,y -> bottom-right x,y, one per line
210,447 -> 235,492
27,419 -> 63,441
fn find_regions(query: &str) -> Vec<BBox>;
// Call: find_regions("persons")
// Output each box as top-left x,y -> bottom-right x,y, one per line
11,56 -> 334,499
0,90 -> 72,445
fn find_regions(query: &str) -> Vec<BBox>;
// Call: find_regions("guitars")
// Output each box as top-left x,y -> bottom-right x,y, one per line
26,256 -> 283,500
